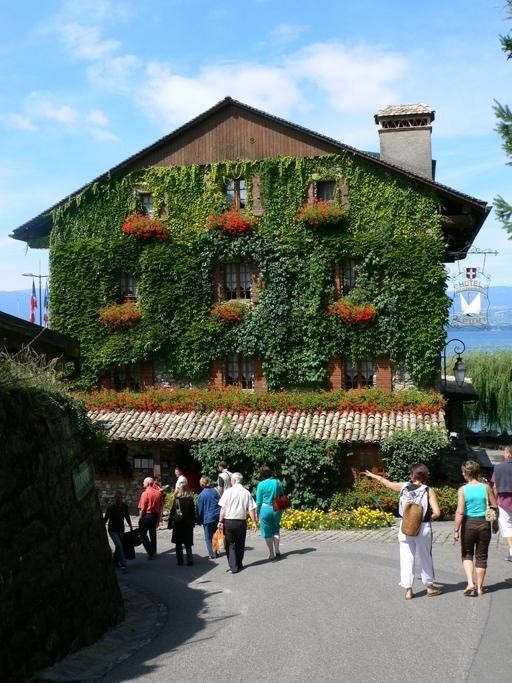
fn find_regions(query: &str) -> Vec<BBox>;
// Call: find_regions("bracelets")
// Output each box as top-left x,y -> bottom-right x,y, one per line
218,520 -> 223,522
455,529 -> 460,533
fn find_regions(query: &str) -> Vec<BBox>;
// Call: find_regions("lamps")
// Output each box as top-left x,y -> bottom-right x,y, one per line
442,337 -> 469,388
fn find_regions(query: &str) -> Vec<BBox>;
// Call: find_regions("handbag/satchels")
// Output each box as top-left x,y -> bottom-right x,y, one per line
273,495 -> 291,511
125,528 -> 143,546
485,508 -> 497,521
174,509 -> 182,521
212,527 -> 226,554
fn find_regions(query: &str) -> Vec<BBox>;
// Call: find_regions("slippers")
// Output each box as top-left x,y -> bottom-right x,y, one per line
428,588 -> 442,597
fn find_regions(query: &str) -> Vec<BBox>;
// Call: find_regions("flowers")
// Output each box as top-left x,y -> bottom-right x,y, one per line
329,300 -> 375,323
93,303 -> 144,330
295,194 -> 350,228
201,210 -> 259,236
209,298 -> 247,324
124,214 -> 169,243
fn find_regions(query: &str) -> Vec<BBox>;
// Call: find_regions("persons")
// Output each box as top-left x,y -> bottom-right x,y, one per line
168,483 -> 195,566
255,467 -> 286,561
360,463 -> 443,600
452,458 -> 498,597
174,467 -> 188,489
196,475 -> 221,559
491,444 -> 511,561
217,460 -> 232,495
217,471 -> 257,573
137,475 -> 167,561
103,490 -> 133,574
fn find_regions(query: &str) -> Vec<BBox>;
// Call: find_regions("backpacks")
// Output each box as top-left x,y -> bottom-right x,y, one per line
401,491 -> 423,536
